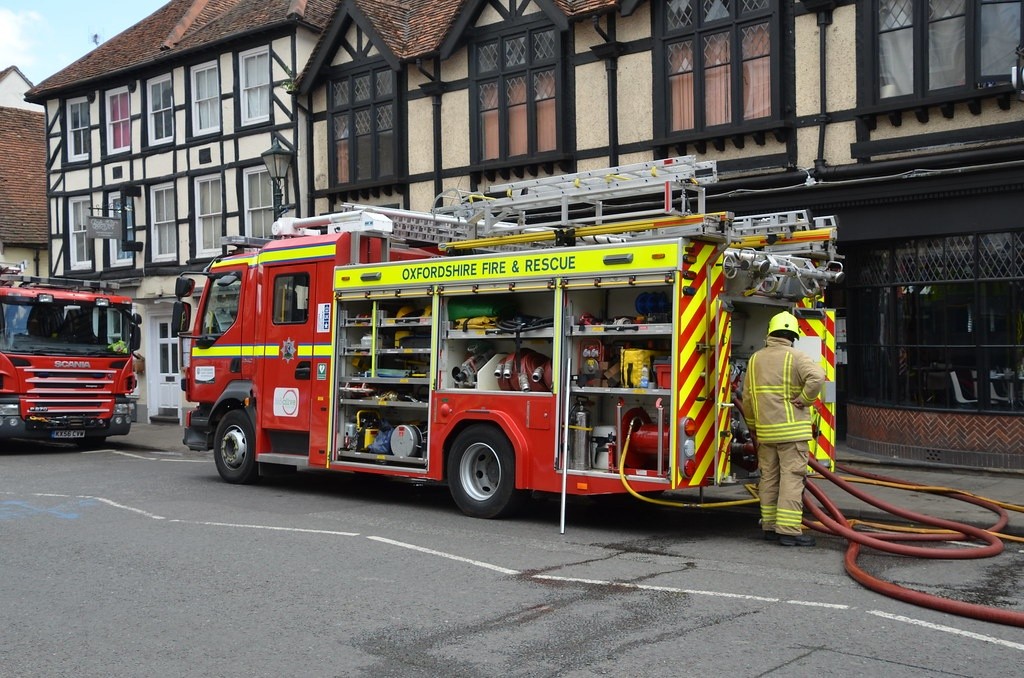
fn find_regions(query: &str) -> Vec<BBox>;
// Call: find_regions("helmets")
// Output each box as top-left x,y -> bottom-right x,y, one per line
766,311 -> 800,340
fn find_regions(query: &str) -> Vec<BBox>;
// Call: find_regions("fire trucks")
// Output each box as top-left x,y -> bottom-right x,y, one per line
172,155 -> 843,519
0,260 -> 141,450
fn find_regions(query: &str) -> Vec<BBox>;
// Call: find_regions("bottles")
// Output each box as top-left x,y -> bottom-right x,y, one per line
344,432 -> 350,447
641,364 -> 648,388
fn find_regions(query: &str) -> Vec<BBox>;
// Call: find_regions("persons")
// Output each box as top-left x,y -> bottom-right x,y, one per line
741,310 -> 826,548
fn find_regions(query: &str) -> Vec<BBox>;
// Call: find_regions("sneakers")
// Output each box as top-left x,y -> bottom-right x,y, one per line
764,531 -> 816,546
